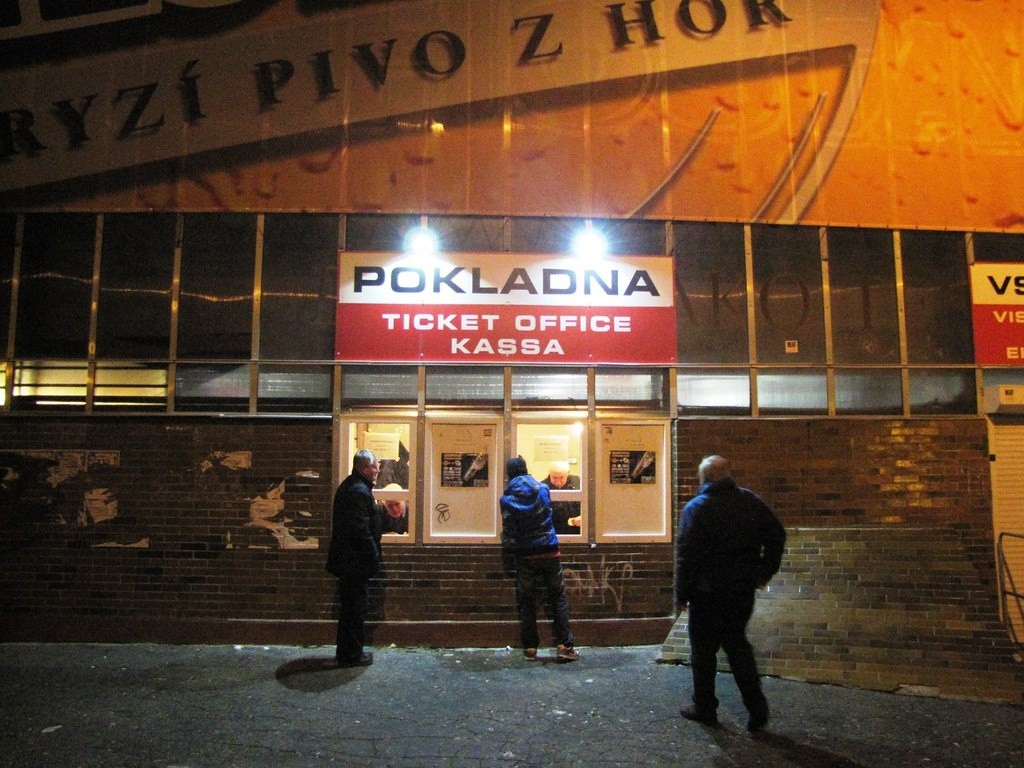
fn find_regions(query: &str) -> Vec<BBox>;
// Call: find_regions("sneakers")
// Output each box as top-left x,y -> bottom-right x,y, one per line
524,647 -> 537,661
556,643 -> 579,661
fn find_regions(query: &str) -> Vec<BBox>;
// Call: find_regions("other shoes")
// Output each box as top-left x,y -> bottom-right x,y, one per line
336,652 -> 373,668
681,703 -> 718,724
745,714 -> 768,730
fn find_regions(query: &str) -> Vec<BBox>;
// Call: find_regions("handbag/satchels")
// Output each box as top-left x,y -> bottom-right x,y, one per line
325,536 -> 371,582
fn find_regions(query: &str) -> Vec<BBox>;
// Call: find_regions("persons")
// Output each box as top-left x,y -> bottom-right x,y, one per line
376,483 -> 409,535
539,462 -> 581,535
499,458 -> 579,661
325,450 -> 383,664
673,455 -> 785,729
